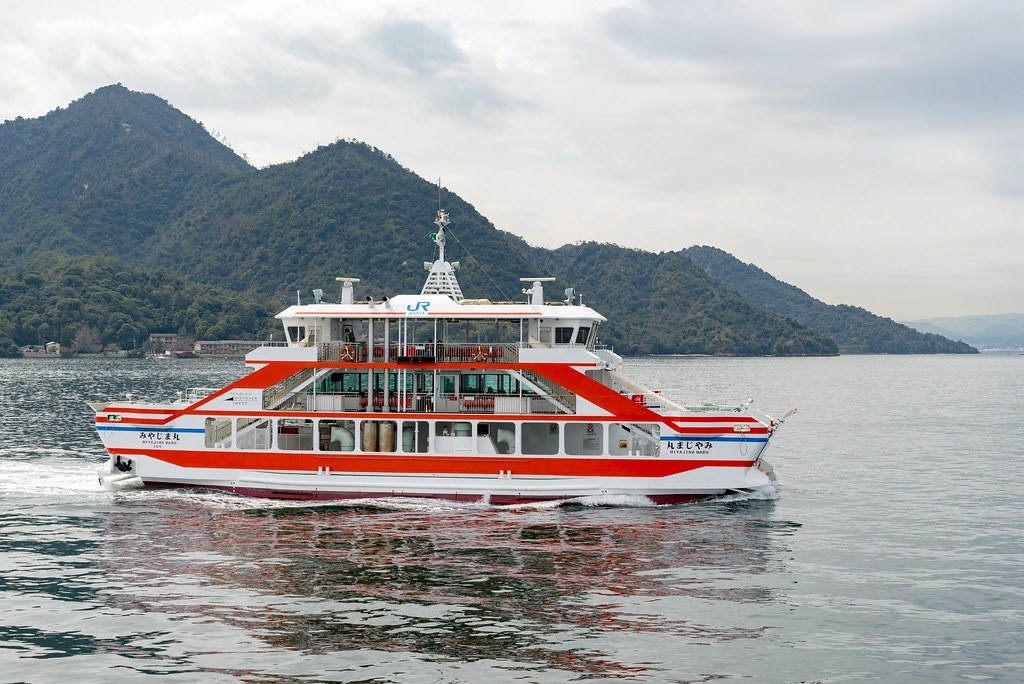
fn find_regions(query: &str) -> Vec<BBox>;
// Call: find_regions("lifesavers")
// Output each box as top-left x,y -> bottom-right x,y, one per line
340,345 -> 355,361
472,346 -> 488,363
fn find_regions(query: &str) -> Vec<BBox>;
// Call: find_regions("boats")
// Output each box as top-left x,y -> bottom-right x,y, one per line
84,176 -> 794,510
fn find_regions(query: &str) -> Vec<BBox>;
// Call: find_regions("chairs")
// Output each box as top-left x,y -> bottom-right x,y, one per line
359,397 -> 411,412
464,396 -> 494,412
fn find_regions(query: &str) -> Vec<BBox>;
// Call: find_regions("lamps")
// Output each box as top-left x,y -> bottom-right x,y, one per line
562,288 -> 576,306
366,296 -> 374,309
382,296 -> 390,308
312,288 -> 323,304
